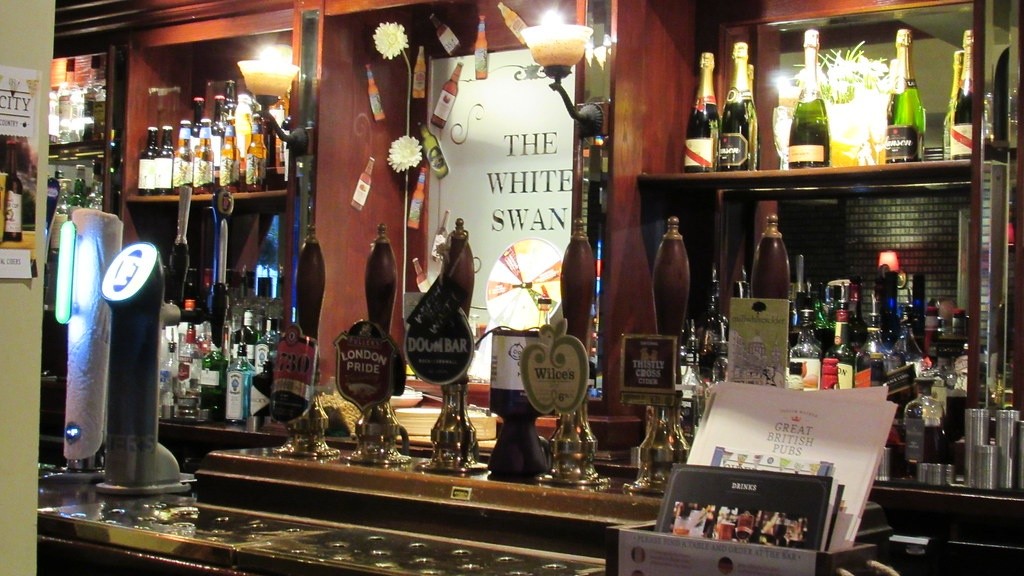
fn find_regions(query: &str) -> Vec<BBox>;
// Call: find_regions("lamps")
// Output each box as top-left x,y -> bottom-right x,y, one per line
520,11 -> 604,136
237,46 -> 308,157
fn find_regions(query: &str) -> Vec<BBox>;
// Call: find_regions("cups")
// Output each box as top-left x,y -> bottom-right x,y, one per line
671,504 -> 808,552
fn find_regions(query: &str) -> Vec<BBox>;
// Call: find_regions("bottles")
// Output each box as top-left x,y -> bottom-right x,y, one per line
160,308 -> 279,426
678,281 -> 968,463
137,80 -> 291,197
50,56 -> 114,148
720,42 -> 761,172
787,27 -> 830,169
942,30 -> 987,160
351,2 -> 528,293
47,161 -> 103,260
681,51 -> 721,174
880,28 -> 926,164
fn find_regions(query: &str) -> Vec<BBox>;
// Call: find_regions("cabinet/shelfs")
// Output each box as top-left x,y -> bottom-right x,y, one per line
37,0 -> 1024,576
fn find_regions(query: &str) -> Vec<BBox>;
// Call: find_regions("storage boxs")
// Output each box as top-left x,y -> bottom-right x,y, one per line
605,519 -> 877,576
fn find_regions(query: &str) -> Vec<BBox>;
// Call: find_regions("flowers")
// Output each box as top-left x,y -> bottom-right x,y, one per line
387,135 -> 423,173
788,41 -> 895,164
372,21 -> 409,60
